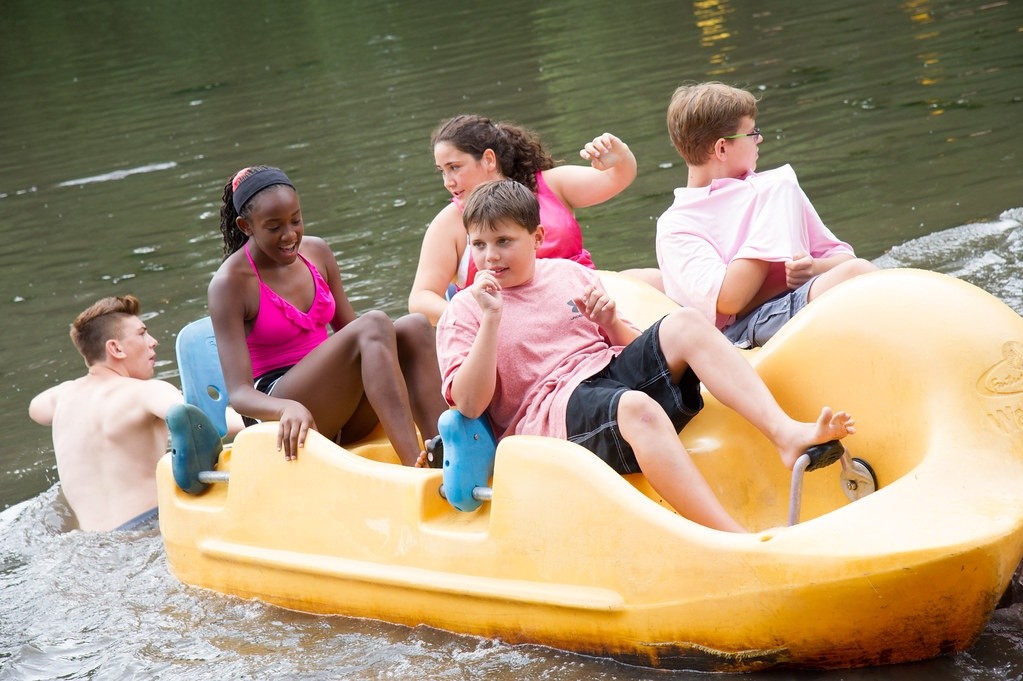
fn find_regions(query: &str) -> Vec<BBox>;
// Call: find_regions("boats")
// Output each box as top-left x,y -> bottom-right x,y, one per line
156,268 -> 1022,681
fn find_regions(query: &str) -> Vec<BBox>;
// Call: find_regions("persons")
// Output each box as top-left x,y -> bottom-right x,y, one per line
408,115 -> 667,327
208,167 -> 449,468
29,295 -> 246,534
435,179 -> 855,533
656,81 -> 880,350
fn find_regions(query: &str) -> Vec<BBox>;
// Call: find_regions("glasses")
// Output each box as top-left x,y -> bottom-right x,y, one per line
714,127 -> 761,149
